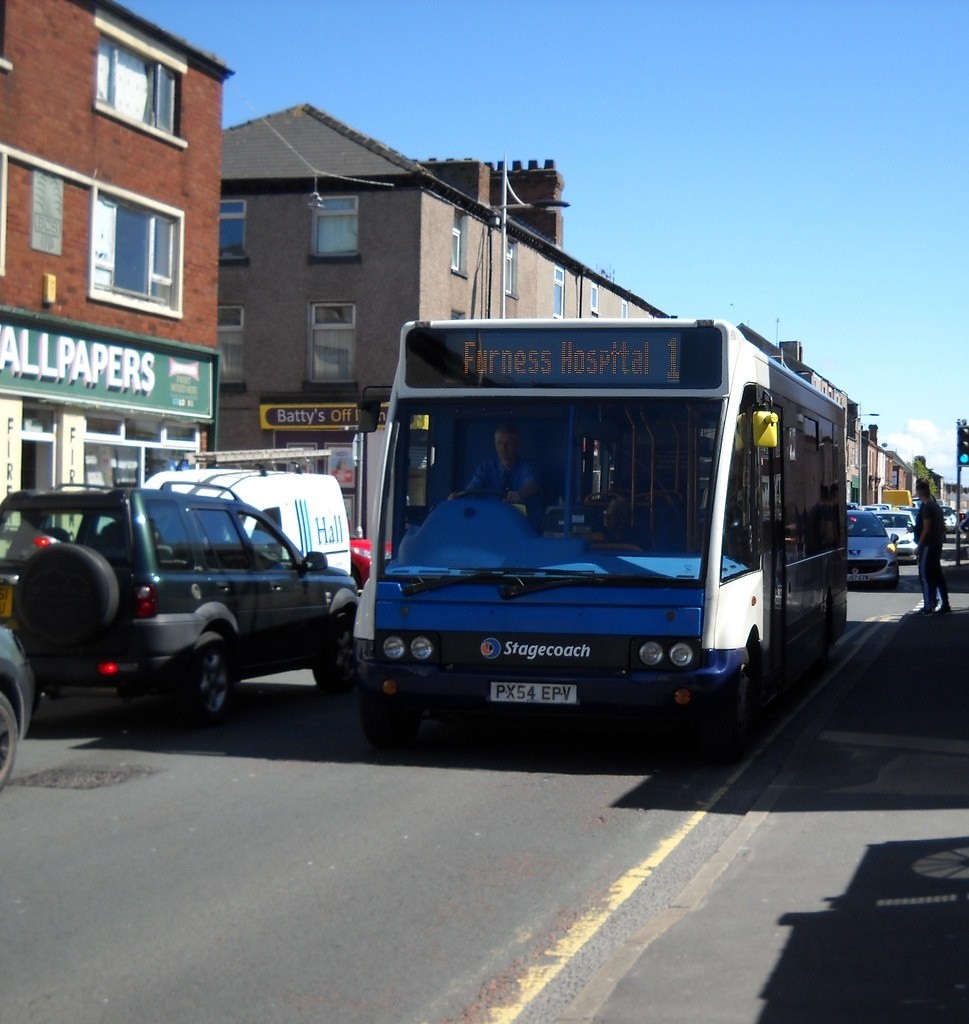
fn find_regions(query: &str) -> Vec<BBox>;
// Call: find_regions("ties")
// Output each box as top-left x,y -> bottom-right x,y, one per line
503,468 -> 512,497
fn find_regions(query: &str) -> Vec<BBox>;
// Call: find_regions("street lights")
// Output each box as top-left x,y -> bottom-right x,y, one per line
499,152 -> 568,319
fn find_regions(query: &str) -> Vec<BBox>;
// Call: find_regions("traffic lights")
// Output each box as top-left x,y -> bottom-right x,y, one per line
957,426 -> 969,466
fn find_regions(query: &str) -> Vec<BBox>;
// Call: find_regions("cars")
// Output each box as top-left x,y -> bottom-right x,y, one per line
939,507 -> 956,533
870,510 -> 918,564
0,628 -> 35,789
846,511 -> 898,586
848,504 -> 919,519
349,533 -> 393,587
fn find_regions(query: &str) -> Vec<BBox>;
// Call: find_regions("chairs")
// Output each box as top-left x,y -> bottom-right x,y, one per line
518,461 -> 687,554
883,518 -> 894,527
97,522 -> 160,569
895,518 -> 907,526
43,526 -> 70,543
174,543 -> 213,568
212,543 -> 282,571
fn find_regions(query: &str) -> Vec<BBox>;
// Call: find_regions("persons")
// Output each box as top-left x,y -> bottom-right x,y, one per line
914,481 -> 952,616
905,478 -> 943,611
448,424 -> 540,529
957,517 -> 969,542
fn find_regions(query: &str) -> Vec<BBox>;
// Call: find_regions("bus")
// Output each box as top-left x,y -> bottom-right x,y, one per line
366,317 -> 849,726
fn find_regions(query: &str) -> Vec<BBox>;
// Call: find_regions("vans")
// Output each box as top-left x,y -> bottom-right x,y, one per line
882,490 -> 912,506
142,468 -> 352,579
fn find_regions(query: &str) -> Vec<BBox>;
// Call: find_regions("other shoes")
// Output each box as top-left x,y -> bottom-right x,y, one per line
935,608 -> 952,614
913,608 -> 934,616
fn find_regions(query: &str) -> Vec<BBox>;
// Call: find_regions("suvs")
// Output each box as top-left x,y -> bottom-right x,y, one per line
0,478 -> 359,730
